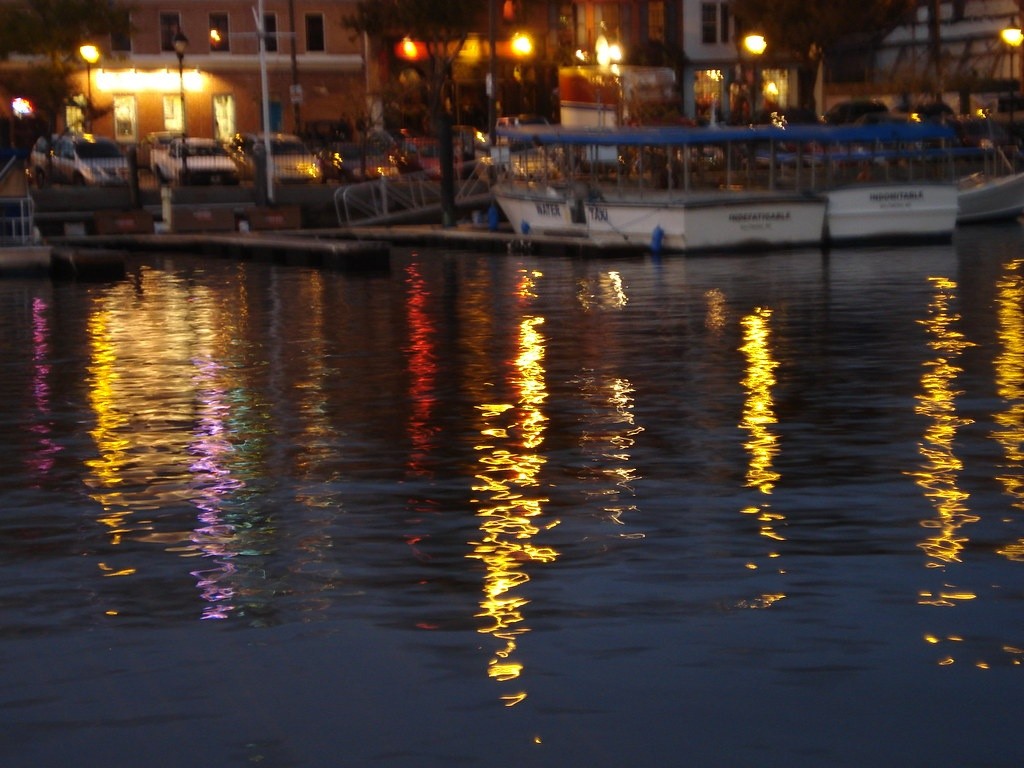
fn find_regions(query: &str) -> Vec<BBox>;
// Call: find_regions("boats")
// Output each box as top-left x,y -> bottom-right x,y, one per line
954,148 -> 1024,227
487,117 -> 829,253
806,125 -> 968,246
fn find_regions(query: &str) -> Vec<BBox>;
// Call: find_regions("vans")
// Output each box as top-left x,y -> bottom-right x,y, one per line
33,133 -> 141,186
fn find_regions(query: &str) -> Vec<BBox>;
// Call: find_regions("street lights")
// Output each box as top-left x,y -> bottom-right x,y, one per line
170,30 -> 192,185
80,42 -> 99,136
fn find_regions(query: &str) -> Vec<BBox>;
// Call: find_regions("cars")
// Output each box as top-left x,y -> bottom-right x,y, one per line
227,132 -> 325,185
304,117 -> 485,190
147,130 -> 240,187
